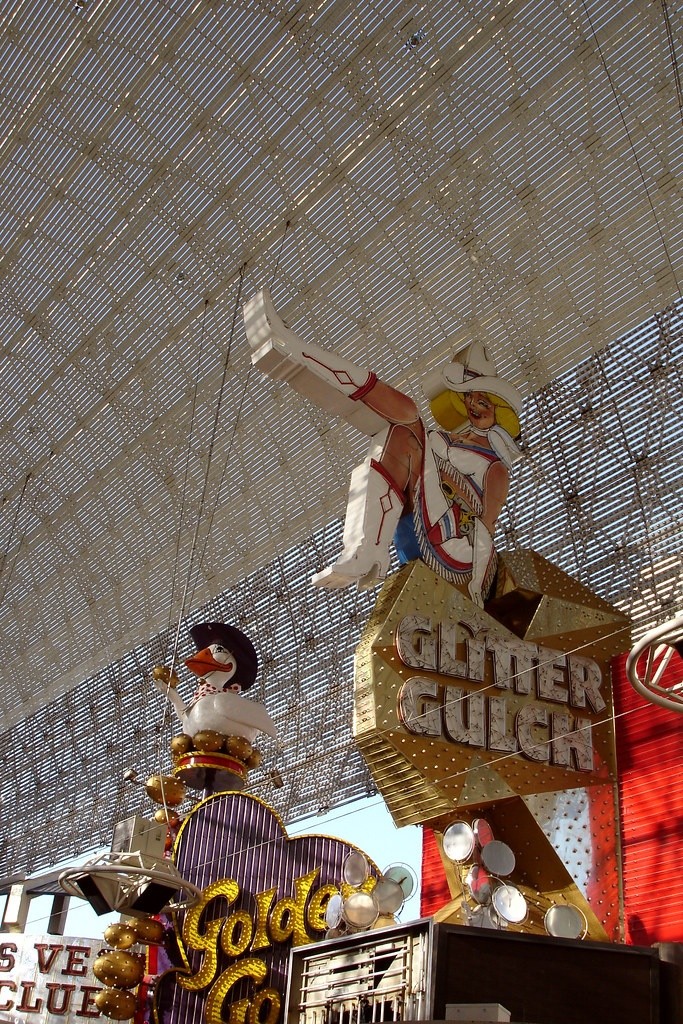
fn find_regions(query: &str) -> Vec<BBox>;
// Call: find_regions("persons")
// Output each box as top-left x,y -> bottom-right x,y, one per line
242,290 -> 523,607
